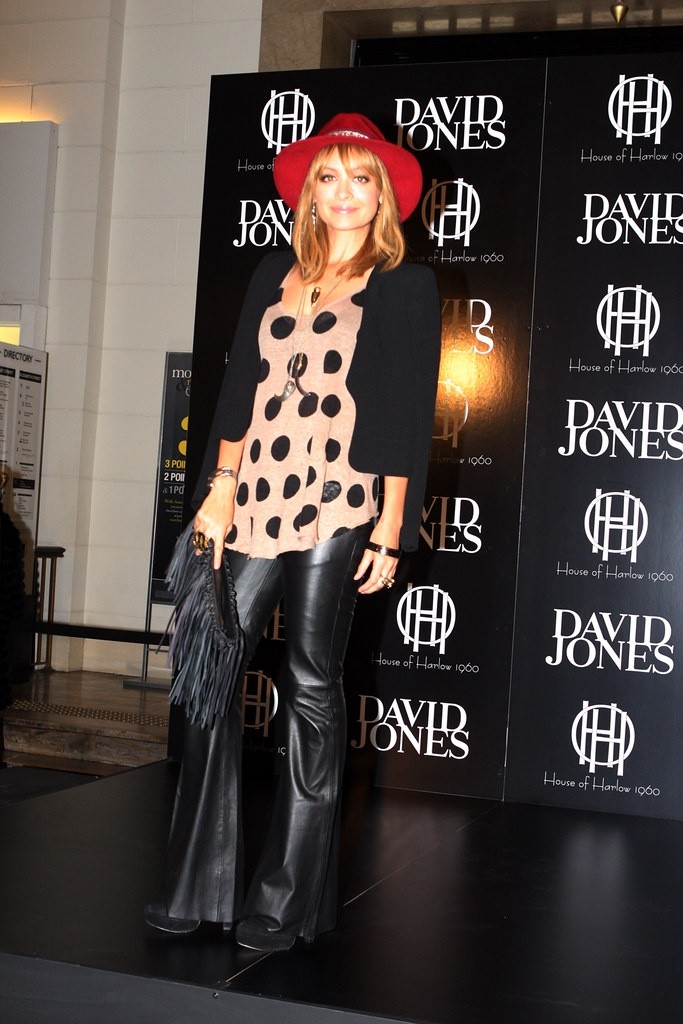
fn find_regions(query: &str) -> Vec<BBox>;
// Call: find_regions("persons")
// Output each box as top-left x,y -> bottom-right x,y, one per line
142,114 -> 442,953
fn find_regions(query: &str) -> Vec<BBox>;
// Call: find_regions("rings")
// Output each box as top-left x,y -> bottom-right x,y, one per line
380,574 -> 395,588
192,531 -> 205,549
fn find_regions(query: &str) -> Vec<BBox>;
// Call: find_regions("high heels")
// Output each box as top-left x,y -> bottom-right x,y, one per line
236,917 -> 315,951
144,901 -> 233,934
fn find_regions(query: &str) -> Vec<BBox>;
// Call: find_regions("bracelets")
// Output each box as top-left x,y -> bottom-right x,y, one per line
364,540 -> 402,559
206,467 -> 238,489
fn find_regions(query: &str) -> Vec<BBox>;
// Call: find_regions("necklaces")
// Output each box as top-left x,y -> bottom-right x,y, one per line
273,273 -> 348,404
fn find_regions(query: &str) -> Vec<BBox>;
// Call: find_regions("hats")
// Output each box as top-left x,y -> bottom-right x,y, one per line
273,113 -> 422,222
